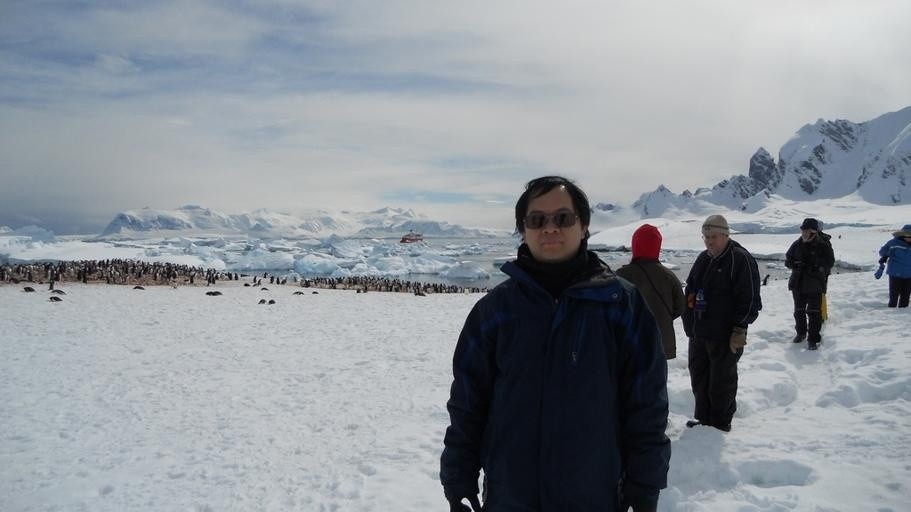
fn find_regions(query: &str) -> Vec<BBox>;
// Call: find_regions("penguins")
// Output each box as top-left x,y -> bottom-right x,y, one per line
762,273 -> 771,286
0,258 -> 289,304
293,275 -> 494,296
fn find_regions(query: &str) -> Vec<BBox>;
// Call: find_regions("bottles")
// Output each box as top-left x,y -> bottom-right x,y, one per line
694,290 -> 704,320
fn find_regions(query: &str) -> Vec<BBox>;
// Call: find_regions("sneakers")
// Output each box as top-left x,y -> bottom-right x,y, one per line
793,327 -> 821,351
686,419 -> 731,432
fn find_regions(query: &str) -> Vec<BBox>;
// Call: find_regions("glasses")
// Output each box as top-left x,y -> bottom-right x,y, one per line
521,211 -> 580,231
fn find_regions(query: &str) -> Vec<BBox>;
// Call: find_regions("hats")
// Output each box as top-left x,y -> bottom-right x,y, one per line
632,224 -> 662,261
701,213 -> 730,236
799,216 -> 824,232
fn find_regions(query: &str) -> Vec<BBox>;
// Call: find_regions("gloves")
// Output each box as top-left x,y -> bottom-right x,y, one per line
729,326 -> 747,354
443,486 -> 481,512
874,264 -> 886,279
621,488 -> 662,512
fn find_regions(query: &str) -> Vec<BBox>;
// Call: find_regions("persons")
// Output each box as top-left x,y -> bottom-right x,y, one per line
875,224 -> 911,310
614,223 -> 684,364
439,171 -> 671,512
679,211 -> 765,435
814,221 -> 835,325
784,216 -> 835,350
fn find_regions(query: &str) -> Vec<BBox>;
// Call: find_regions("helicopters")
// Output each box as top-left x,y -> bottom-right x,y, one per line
399,229 -> 424,243
